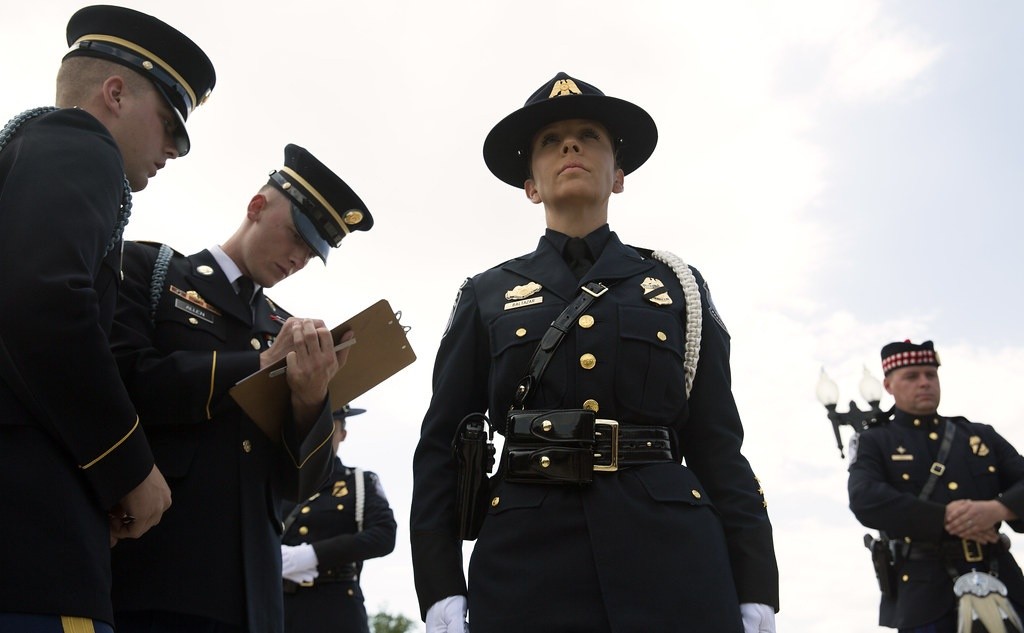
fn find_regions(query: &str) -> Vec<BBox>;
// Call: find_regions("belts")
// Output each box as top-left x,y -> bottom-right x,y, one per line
896,533 -> 1002,562
282,562 -> 359,586
592,419 -> 672,473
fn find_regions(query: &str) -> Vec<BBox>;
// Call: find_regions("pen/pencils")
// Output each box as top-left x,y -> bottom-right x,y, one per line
270,313 -> 285,325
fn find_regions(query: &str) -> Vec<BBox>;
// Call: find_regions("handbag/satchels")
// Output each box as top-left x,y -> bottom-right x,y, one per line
863,535 -> 897,601
449,414 -> 498,540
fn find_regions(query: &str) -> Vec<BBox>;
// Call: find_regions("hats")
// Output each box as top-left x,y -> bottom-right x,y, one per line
332,405 -> 366,420
267,143 -> 374,266
482,72 -> 658,190
881,339 -> 941,376
62,4 -> 217,157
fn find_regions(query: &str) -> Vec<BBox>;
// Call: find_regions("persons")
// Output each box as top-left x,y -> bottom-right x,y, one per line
0,5 -> 216,633
409,71 -> 780,633
109,143 -> 374,632
847,339 -> 1024,633
281,403 -> 398,633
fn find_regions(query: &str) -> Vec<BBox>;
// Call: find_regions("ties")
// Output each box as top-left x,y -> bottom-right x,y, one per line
565,236 -> 593,282
235,275 -> 255,303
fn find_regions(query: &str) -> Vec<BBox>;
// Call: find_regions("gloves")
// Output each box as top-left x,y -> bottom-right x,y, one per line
280,542 -> 319,583
740,603 -> 776,633
426,595 -> 470,633
